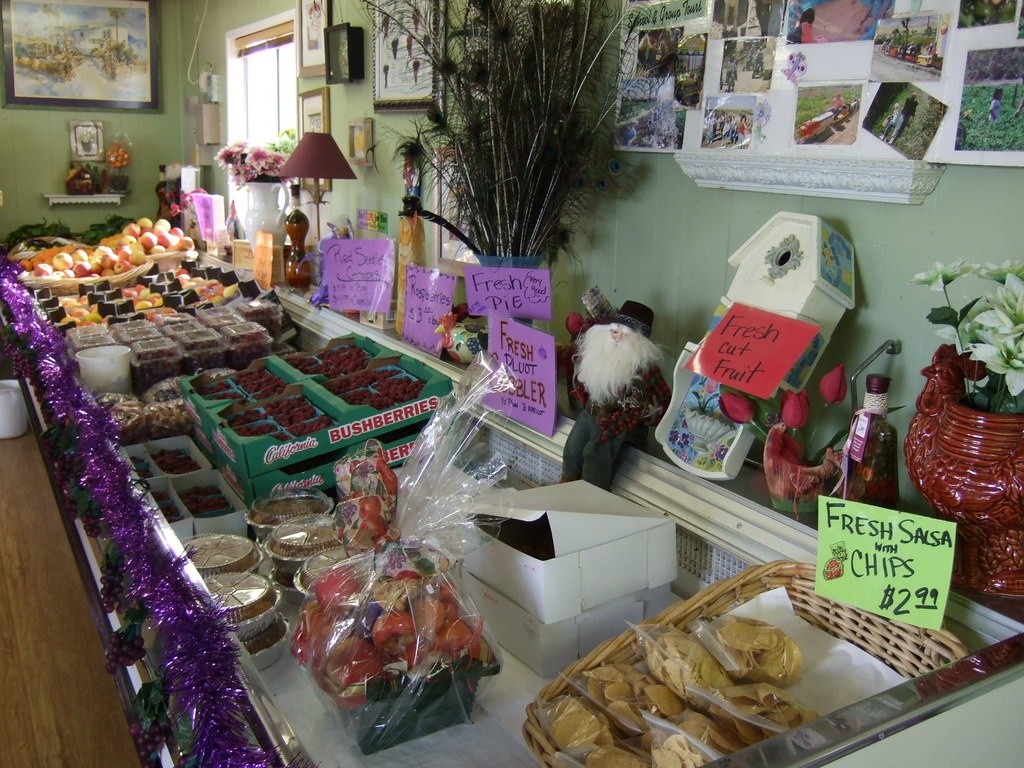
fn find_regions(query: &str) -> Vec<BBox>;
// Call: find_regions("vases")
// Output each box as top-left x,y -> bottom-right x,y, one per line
244,181 -> 290,257
902,344 -> 1024,600
112,175 -> 128,190
471,247 -> 550,325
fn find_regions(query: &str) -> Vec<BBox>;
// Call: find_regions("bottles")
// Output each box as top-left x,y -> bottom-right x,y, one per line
843,372 -> 899,511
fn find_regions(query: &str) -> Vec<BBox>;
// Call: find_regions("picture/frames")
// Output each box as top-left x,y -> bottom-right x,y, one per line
297,86 -> 332,192
372,0 -> 446,114
297,0 -> 333,77
0,0 -> 165,116
348,118 -> 374,167
68,121 -> 105,162
432,147 -> 480,277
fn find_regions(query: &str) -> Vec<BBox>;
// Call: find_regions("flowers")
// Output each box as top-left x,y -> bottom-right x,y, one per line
718,362 -> 905,464
213,127 -> 299,192
908,256 -> 1024,416
106,144 -> 133,175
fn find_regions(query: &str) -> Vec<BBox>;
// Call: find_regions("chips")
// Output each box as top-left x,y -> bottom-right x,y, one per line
537,617 -> 817,768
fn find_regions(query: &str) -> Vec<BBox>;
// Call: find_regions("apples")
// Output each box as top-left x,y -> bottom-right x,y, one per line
314,563 -> 383,691
34,217 -> 237,327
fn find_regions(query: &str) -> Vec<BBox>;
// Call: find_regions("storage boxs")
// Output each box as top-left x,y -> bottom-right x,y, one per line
65,298 -> 458,541
303,650 -> 501,753
459,479 -> 681,680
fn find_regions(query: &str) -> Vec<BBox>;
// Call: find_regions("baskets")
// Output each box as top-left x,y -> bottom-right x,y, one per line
147,251 -> 200,271
18,259 -> 154,296
521,561 -> 969,768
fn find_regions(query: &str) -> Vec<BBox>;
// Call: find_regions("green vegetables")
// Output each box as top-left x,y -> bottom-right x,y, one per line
5,213 -> 136,251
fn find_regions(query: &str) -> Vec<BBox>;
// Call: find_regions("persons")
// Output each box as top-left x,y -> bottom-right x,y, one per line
878,92 -> 917,145
707,111 -> 748,147
881,39 -> 934,62
559,301 -> 670,493
990,88 -> 1004,122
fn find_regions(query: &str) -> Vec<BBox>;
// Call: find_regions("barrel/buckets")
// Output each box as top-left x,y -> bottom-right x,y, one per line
76,345 -> 131,394
0,379 -> 29,439
